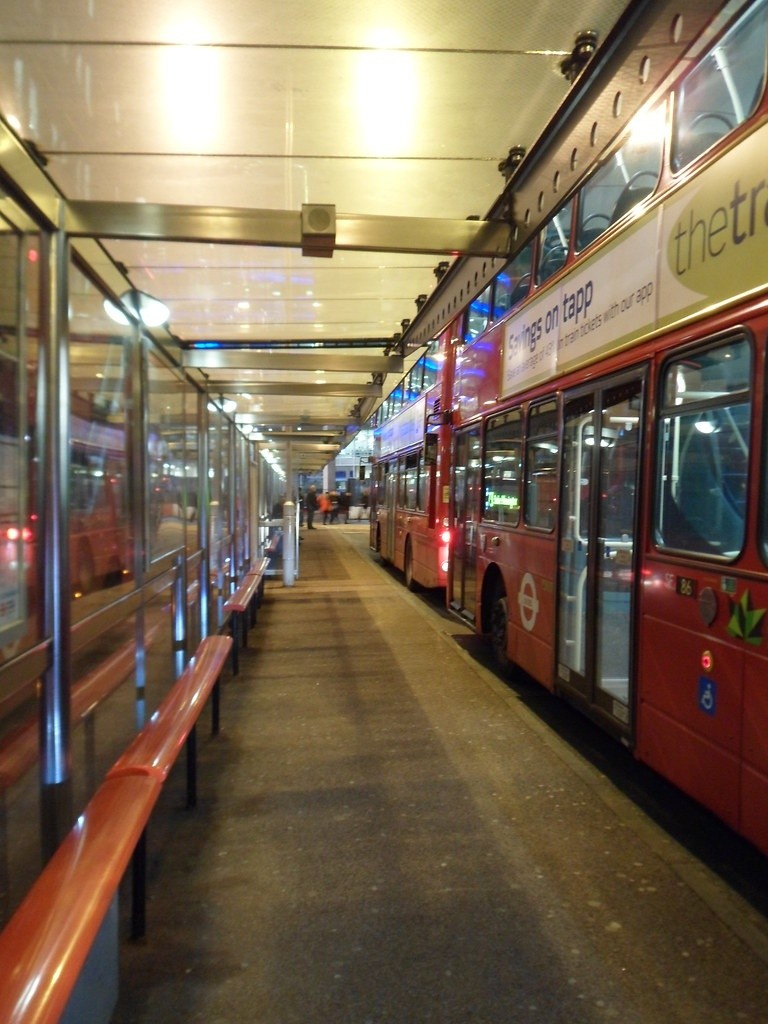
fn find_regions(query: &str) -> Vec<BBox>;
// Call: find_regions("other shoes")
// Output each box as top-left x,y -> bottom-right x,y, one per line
309,527 -> 316,529
323,523 -> 326,525
345,522 -> 349,524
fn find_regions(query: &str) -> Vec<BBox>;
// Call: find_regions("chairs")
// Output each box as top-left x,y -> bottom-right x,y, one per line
509,112 -> 735,307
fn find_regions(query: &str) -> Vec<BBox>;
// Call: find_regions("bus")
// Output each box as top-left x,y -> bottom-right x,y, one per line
0,347 -> 149,639
370,1 -> 768,859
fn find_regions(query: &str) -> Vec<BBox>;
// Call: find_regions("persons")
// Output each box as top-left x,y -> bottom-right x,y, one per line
306,487 -> 317,529
316,491 -> 354,525
298,488 -> 305,540
358,488 -> 371,522
